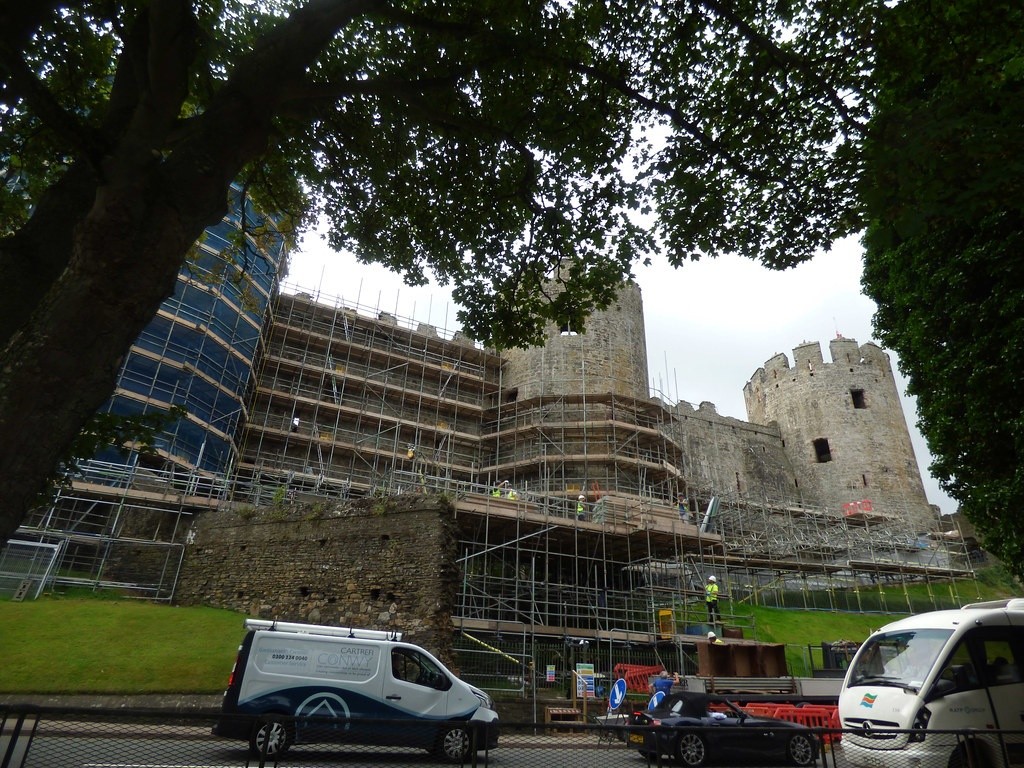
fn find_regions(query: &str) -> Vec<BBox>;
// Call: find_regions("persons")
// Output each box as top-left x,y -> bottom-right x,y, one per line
493,480 -> 517,502
648,670 -> 679,697
392,654 -> 407,681
705,575 -> 721,623
708,632 -> 724,643
675,494 -> 689,524
577,494 -> 587,522
407,444 -> 415,461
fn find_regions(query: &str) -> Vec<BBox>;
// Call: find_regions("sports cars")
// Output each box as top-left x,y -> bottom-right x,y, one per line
624,691 -> 824,768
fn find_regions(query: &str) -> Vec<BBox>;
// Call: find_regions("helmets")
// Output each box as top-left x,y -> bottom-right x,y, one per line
579,495 -> 585,499
709,576 -> 716,582
708,632 -> 715,639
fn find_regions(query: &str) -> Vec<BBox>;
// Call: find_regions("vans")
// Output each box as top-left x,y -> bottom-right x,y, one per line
210,618 -> 501,765
837,597 -> 1024,768
